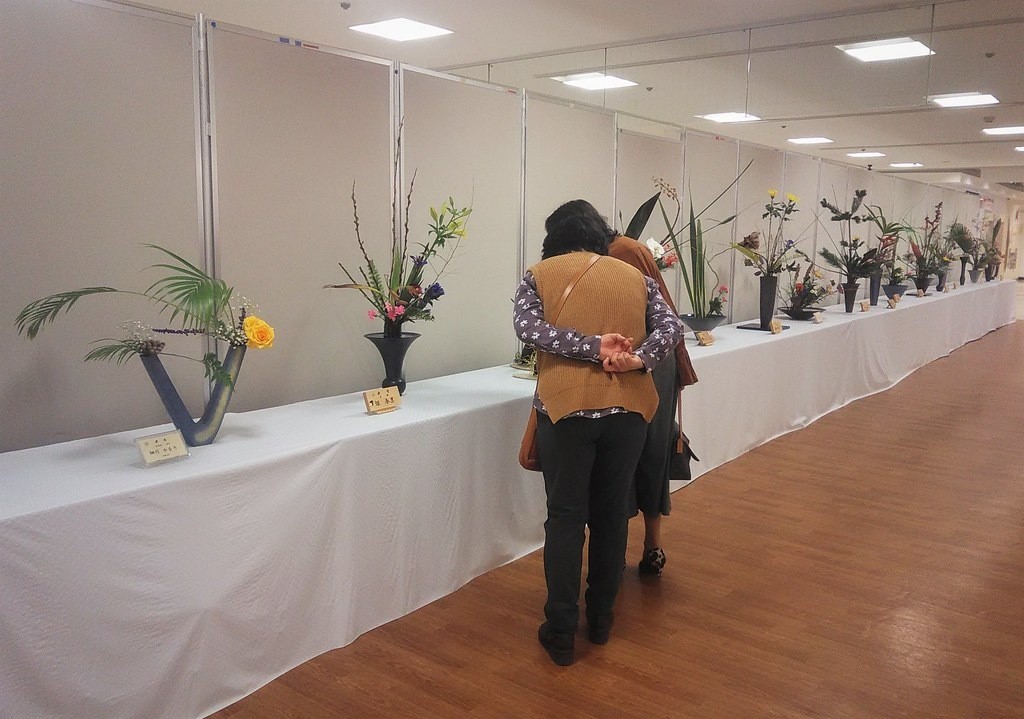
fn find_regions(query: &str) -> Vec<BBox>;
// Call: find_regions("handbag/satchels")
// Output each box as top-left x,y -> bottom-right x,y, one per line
519,407 -> 543,471
669,421 -> 700,481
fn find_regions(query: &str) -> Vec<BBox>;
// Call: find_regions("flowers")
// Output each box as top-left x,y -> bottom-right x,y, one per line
14,243 -> 276,359
777,260 -> 844,307
864,203 -> 896,274
650,176 -> 732,313
902,200 -> 943,269
818,189 -> 877,282
322,113 -> 475,327
933,231 -> 953,262
729,190 -> 808,276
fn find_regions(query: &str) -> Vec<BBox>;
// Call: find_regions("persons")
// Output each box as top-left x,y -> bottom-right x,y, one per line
513,216 -> 683,666
544,200 -> 699,582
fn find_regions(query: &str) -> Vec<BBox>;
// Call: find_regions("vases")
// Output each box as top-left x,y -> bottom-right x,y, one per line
759,276 -> 777,327
936,261 -> 950,291
778,307 -> 826,320
870,275 -> 881,305
679,314 -> 728,341
364,331 -> 422,396
137,344 -> 248,449
881,211 -> 909,299
913,278 -> 935,293
841,282 -> 862,314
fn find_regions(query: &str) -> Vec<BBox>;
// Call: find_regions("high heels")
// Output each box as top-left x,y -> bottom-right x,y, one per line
639,547 -> 666,582
538,621 -> 575,667
587,609 -> 613,644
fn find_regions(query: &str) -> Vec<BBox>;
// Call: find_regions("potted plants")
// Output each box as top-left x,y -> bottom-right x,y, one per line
953,223 -> 969,285
984,244 -> 1002,281
967,236 -> 984,283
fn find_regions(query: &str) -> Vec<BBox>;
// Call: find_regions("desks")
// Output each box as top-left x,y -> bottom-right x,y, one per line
0,278 -> 1019,719
